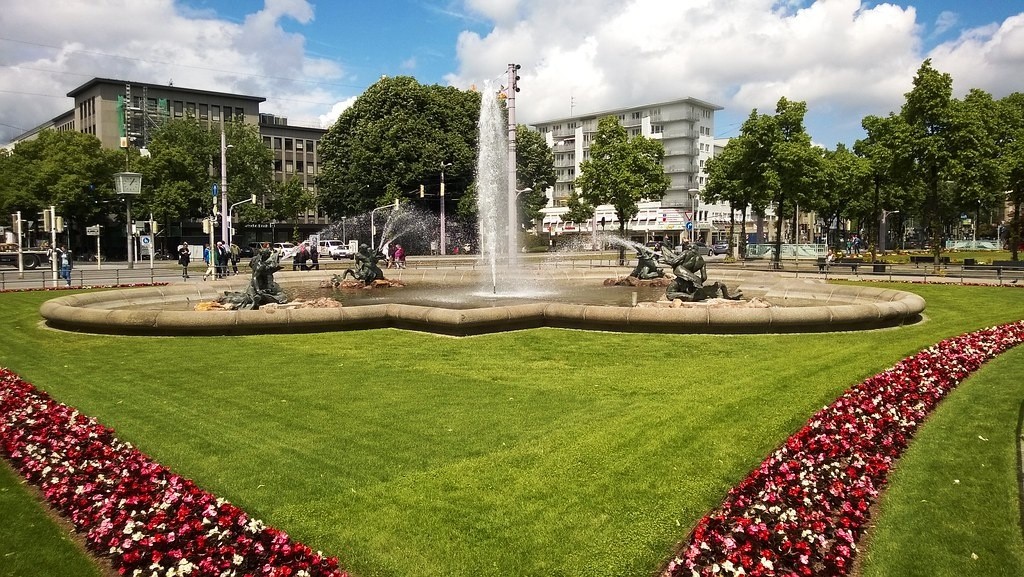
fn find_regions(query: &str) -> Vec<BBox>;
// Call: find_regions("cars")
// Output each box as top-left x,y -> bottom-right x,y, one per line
274,242 -> 297,258
675,242 -> 713,256
71,250 -> 106,262
142,249 -> 174,261
713,239 -> 730,256
238,244 -> 254,258
332,246 -> 355,260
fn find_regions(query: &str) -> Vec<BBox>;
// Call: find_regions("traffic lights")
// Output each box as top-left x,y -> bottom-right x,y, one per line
231,228 -> 238,236
37,210 -> 50,233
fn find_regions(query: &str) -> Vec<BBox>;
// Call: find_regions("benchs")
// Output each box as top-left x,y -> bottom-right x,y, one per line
910,256 -> 951,268
812,257 -> 864,274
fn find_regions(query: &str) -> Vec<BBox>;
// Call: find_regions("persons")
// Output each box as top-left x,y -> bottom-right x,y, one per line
250,249 -> 284,296
682,237 -> 689,251
46,244 -> 52,269
355,243 -> 383,284
262,243 -> 270,253
292,241 -> 319,269
666,243 -> 708,302
847,235 -> 862,254
179,242 -> 192,278
60,245 -> 74,286
387,243 -> 406,268
825,249 -> 837,272
203,241 -> 241,280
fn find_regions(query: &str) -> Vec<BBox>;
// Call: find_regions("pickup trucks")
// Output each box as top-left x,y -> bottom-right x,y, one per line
0,244 -> 50,269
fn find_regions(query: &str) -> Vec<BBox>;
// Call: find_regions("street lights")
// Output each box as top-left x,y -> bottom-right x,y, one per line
879,210 -> 900,252
687,187 -> 700,242
221,145 -> 236,249
441,163 -> 452,256
341,217 -> 346,243
508,188 -> 535,264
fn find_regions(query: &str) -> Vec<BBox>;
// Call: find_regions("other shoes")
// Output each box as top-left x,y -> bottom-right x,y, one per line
183,275 -> 188,278
66,284 -> 69,287
233,270 -> 238,276
203,275 -> 207,280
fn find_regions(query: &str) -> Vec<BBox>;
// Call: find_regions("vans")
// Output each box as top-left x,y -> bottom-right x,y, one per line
247,242 -> 272,256
318,239 -> 345,257
